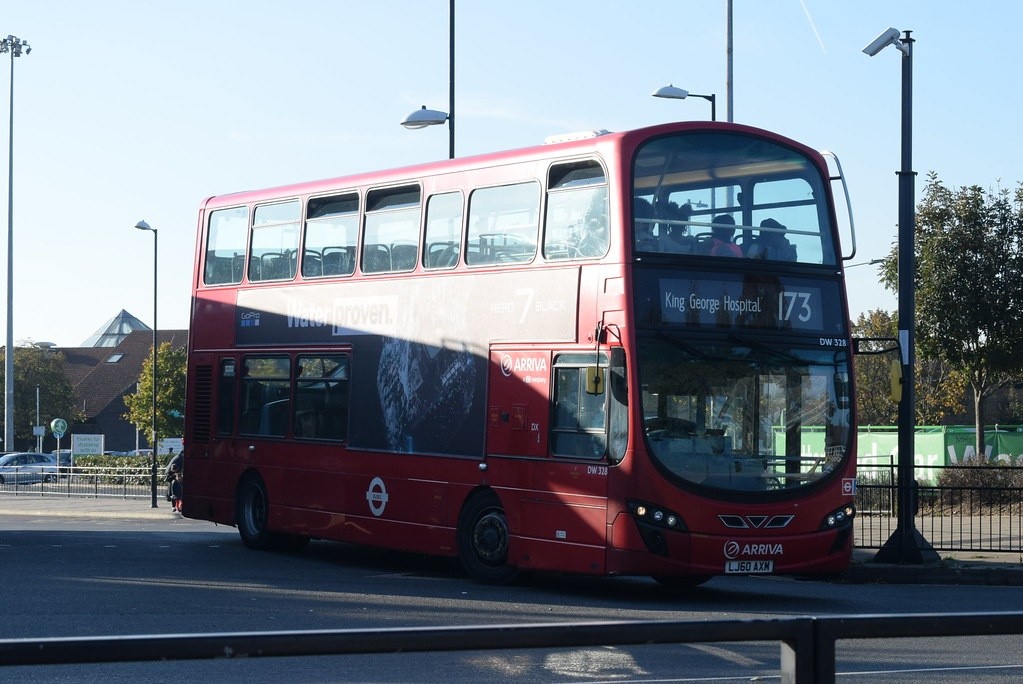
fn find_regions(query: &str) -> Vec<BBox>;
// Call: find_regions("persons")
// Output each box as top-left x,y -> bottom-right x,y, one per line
165,451 -> 183,502
632,197 -> 797,263
167,464 -> 179,512
171,473 -> 183,512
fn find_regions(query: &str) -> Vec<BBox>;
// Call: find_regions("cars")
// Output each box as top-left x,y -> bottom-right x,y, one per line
0,452 -> 62,485
50,448 -> 153,461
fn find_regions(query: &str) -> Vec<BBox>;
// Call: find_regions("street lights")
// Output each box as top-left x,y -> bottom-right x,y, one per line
651,84 -> 716,226
400,105 -> 455,160
134,219 -> 158,509
0,34 -> 34,455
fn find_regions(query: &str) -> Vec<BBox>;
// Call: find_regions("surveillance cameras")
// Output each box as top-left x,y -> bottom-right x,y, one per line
862,27 -> 900,56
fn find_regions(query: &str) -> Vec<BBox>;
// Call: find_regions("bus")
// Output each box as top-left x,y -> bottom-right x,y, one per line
180,121 -> 906,592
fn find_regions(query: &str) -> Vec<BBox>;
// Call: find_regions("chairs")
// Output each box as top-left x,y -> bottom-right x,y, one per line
259,397 -> 290,437
203,234 -> 760,286
295,410 -> 319,439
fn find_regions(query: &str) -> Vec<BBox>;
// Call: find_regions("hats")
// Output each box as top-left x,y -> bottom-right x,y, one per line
759,218 -> 787,236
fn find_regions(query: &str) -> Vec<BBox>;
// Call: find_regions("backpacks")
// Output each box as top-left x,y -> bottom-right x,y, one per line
710,237 -> 743,258
